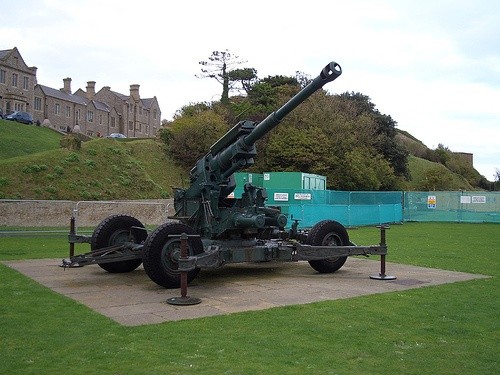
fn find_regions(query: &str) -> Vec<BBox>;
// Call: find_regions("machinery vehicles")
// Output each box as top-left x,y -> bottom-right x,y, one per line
65,61 -> 353,289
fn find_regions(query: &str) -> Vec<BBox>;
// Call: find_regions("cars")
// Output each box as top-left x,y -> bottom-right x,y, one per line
109,133 -> 126,139
4,111 -> 34,126
